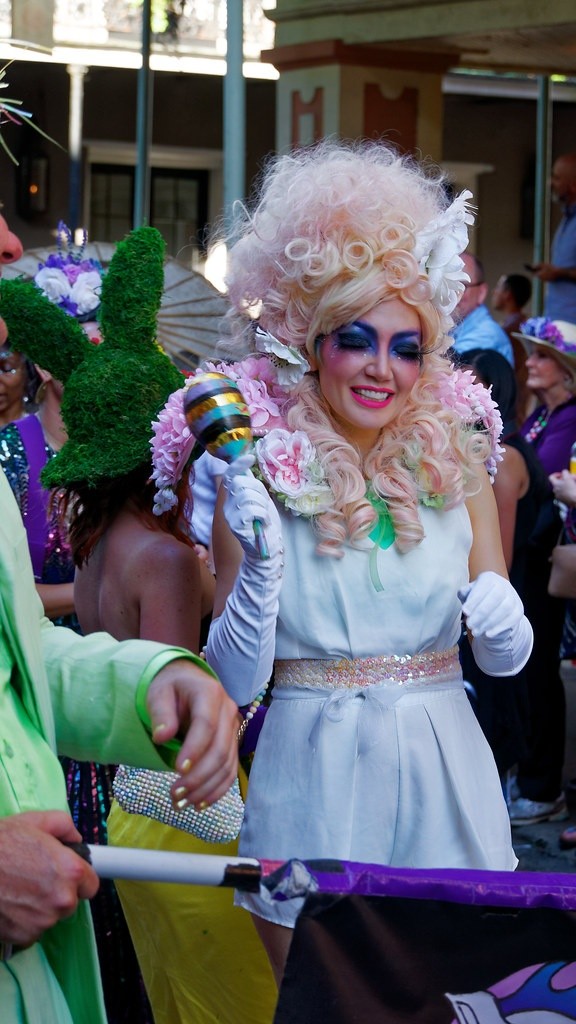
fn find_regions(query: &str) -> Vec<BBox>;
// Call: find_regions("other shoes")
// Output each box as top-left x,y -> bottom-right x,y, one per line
560,822 -> 576,846
509,793 -> 570,827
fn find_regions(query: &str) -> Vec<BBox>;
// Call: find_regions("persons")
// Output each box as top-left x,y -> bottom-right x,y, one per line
3,140 -> 573,1020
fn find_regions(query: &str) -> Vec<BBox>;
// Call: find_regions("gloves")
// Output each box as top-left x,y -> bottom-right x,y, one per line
205,454 -> 281,706
456,571 -> 533,678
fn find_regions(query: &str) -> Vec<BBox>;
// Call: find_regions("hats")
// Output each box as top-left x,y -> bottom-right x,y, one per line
509,318 -> 576,380
0,225 -> 186,487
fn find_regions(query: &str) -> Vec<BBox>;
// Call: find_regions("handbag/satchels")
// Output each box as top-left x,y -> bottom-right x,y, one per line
548,506 -> 576,599
113,644 -> 268,840
